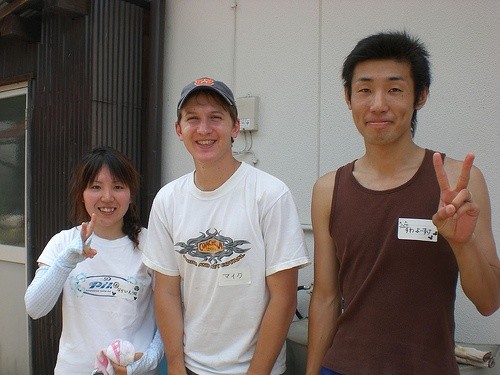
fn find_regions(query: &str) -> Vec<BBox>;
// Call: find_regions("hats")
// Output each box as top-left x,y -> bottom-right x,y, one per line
175,78 -> 238,119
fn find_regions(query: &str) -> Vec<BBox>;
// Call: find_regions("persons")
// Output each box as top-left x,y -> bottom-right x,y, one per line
302,29 -> 498,375
25,147 -> 167,375
141,77 -> 308,375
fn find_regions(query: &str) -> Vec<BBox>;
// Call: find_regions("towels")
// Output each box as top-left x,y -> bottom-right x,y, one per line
91,337 -> 135,375
